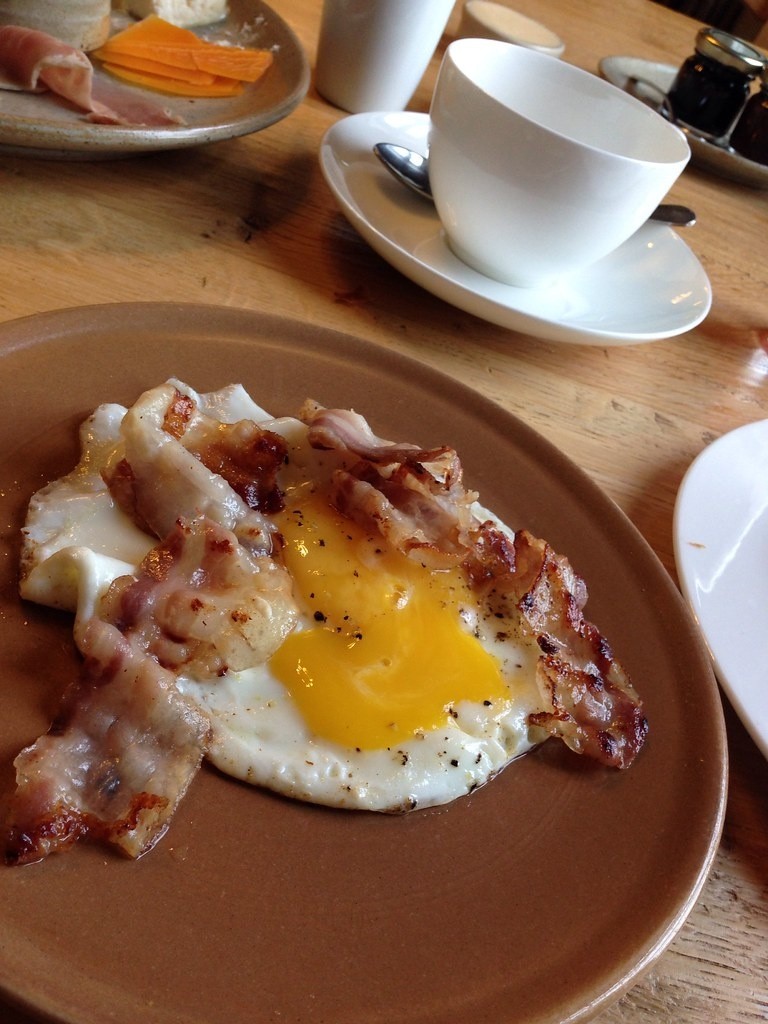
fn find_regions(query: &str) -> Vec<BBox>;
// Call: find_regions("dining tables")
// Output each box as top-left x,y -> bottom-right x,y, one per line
0,0 -> 768,1024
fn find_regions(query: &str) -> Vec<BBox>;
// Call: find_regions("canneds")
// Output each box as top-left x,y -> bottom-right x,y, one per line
659,28 -> 768,170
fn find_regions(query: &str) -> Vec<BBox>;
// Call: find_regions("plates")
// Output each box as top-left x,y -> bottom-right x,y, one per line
597,57 -> 768,187
0,0 -> 310,151
320,112 -> 712,344
672,420 -> 768,753
0,305 -> 727,1024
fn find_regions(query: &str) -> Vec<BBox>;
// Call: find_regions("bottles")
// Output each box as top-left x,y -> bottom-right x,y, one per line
729,61 -> 768,167
660,29 -> 764,144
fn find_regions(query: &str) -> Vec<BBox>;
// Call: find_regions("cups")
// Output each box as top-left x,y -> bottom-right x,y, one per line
454,1 -> 565,59
427,39 -> 692,288
314,0 -> 455,114
0,0 -> 111,52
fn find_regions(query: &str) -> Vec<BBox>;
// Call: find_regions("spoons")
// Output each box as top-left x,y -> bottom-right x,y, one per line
372,141 -> 696,226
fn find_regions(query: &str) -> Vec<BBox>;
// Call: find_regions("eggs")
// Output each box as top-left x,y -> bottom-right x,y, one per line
16,383 -> 566,811
